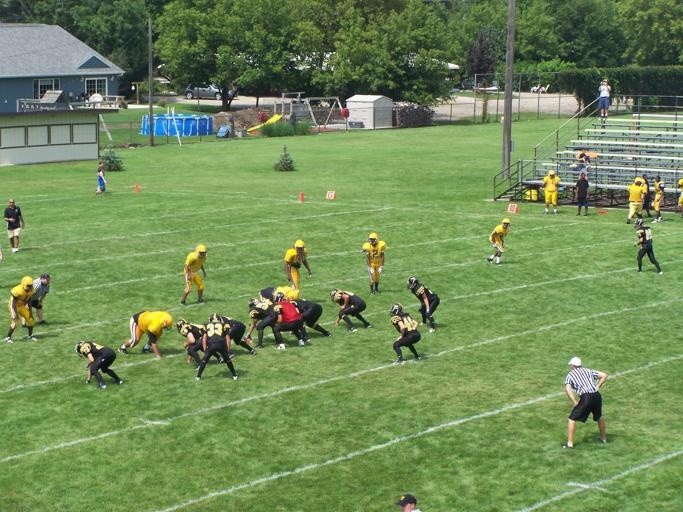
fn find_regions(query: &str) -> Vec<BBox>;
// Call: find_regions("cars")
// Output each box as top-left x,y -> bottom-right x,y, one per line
184,82 -> 237,101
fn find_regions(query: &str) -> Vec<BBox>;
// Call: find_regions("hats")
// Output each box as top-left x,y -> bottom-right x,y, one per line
395,493 -> 416,506
568,356 -> 582,366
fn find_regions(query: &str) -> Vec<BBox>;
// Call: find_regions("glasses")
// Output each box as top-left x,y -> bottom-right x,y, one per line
9,201 -> 15,203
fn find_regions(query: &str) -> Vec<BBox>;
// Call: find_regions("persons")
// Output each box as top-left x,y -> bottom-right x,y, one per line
406,274 -> 439,333
4,198 -> 25,252
244,287 -> 331,351
0,243 -> 3,261
393,494 -> 421,512
117,310 -> 174,359
598,79 -> 611,118
74,340 -> 123,390
541,148 -> 682,224
5,276 -> 37,343
96,161 -> 106,194
359,233 -> 387,295
22,273 -> 50,328
632,218 -> 663,276
625,96 -> 632,112
330,287 -> 372,333
176,313 -> 257,381
179,244 -> 207,306
562,357 -> 608,449
486,218 -> 510,266
283,239 -> 311,291
390,304 -> 423,364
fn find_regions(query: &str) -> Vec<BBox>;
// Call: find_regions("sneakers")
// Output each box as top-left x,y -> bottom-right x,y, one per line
40,320 -> 47,324
658,217 -> 663,222
142,345 -> 151,354
420,322 -> 427,326
95,383 -> 107,389
27,335 -> 37,341
414,355 -> 421,360
185,322 -> 378,382
429,328 -> 436,333
198,300 -> 206,304
15,247 -> 19,251
179,300 -> 186,306
3,336 -> 13,344
486,257 -> 493,263
393,360 -> 404,365
112,379 -> 122,384
656,269 -> 663,274
635,269 -> 642,272
373,289 -> 378,294
117,347 -> 127,356
370,291 -> 375,296
626,219 -> 630,225
495,262 -> 502,267
598,438 -> 607,444
544,209 -> 548,215
560,443 -> 573,448
12,248 -> 15,252
651,218 -> 658,223
553,209 -> 558,215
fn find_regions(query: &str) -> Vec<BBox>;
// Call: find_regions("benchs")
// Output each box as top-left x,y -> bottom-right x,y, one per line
537,120 -> 683,186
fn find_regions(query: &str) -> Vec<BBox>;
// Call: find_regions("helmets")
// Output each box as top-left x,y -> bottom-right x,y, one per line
196,245 -> 206,257
176,319 -> 185,331
634,177 -> 642,185
548,170 -> 555,179
390,304 -> 402,315
209,312 -> 220,323
295,239 -> 305,253
75,341 -> 85,356
502,218 -> 511,229
368,233 -> 378,245
634,218 -> 643,229
248,297 -> 258,310
163,317 -> 174,331
21,276 -> 33,290
407,276 -> 418,289
274,292 -> 285,302
330,288 -> 338,300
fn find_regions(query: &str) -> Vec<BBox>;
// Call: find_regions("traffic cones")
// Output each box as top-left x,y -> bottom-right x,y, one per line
135,183 -> 140,192
298,192 -> 304,202
